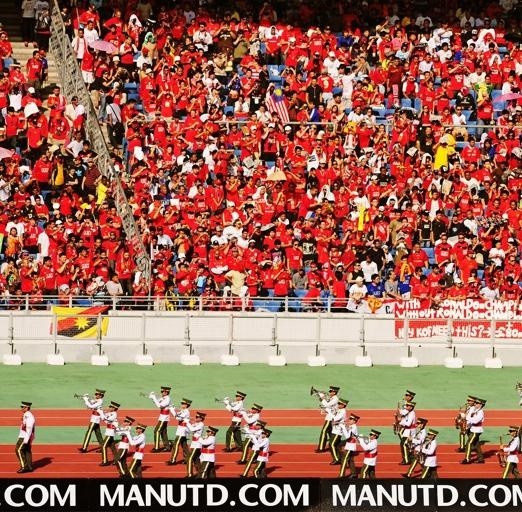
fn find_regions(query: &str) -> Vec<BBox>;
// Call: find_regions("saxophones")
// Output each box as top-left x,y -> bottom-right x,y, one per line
394,397 -> 431,464
495,432 -> 509,466
454,402 -> 474,438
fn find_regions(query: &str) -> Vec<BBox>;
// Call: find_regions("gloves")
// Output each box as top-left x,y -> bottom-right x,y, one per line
97,408 -> 103,414
239,408 -> 246,415
184,418 -> 190,424
339,419 -> 346,429
325,408 -> 331,414
149,393 -> 156,400
83,394 -> 89,402
357,434 -> 366,443
170,407 -> 175,413
319,393 -> 325,398
194,433 -> 201,439
224,397 -> 231,405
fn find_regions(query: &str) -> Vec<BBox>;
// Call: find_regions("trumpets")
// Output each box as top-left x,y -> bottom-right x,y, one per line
74,391 -> 136,438
309,387 -> 370,445
138,389 -> 211,440
213,394 -> 262,448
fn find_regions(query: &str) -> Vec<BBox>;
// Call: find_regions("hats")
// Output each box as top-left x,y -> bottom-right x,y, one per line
416,418 -> 428,424
405,390 -> 416,397
256,420 -> 267,427
406,402 -> 416,407
252,404 -> 263,411
262,429 -> 272,436
109,401 -> 120,408
136,423 -> 147,430
236,391 -> 246,398
21,401 -> 32,407
329,386 -> 340,392
95,389 -> 106,395
125,416 -> 135,423
160,387 -> 171,392
337,398 -> 349,406
369,429 -> 381,437
206,426 -> 218,433
196,412 -> 206,419
349,413 -> 360,422
427,428 -> 439,436
181,398 -> 192,406
508,426 -> 519,431
467,396 -> 487,405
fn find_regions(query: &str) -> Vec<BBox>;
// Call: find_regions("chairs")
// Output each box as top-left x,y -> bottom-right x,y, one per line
0,30 -> 521,313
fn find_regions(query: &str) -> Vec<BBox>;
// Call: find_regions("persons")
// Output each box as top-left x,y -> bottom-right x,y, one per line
114,416 -> 135,478
220,390 -> 272,478
99,401 -> 120,467
456,395 -> 477,452
126,422 -> 147,479
501,425 -> 522,479
315,384 -> 380,480
78,388 -> 106,453
460,398 -> 487,463
148,387 -> 219,479
0,0 -> 521,314
517,384 -> 522,453
15,400 -> 35,474
396,389 -> 439,480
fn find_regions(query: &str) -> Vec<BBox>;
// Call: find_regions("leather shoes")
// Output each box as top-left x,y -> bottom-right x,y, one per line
163,448 -> 170,452
79,448 -> 86,453
399,460 -> 410,465
99,463 -> 108,466
315,449 -> 326,452
151,448 -> 161,453
96,450 -> 102,453
236,459 -> 246,464
18,468 -> 32,473
456,448 -> 484,464
252,459 -> 258,464
329,460 -> 341,465
167,461 -> 175,465
400,472 -> 410,478
222,447 -> 243,452
182,461 -> 187,464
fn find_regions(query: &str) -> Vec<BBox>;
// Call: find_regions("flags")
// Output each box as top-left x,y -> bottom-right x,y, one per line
51,307 -> 109,338
